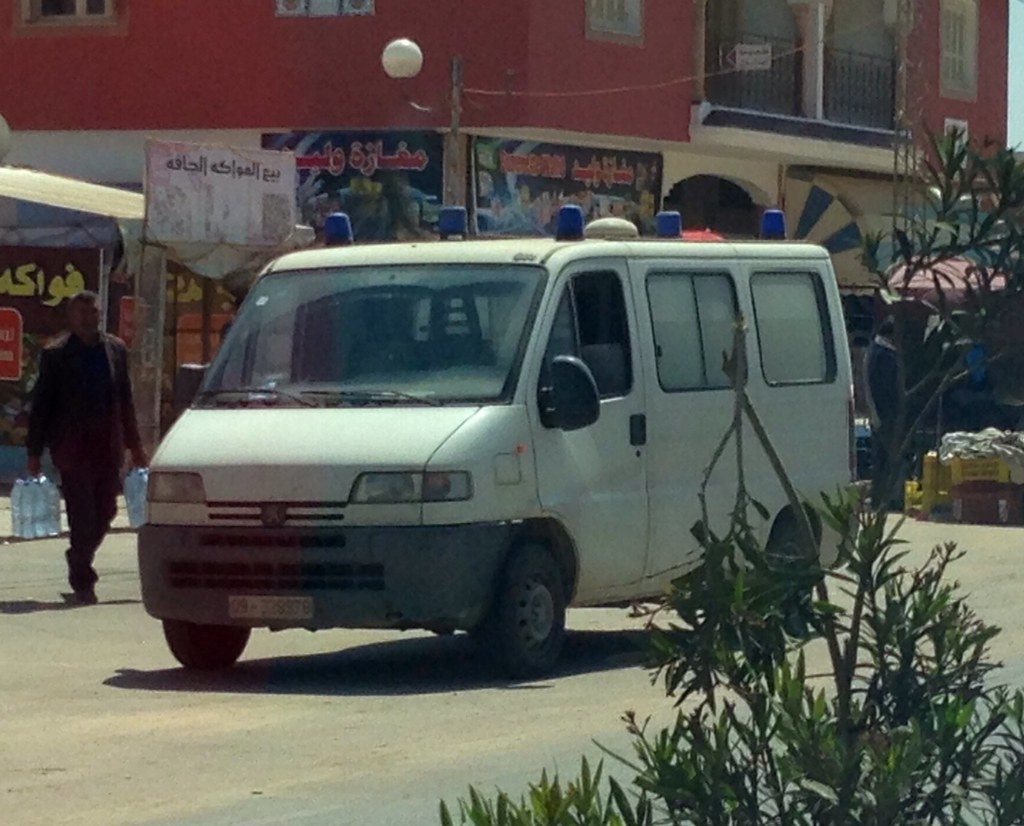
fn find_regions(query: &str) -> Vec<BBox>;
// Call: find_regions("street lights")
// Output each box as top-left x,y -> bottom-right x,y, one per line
381,34 -> 471,239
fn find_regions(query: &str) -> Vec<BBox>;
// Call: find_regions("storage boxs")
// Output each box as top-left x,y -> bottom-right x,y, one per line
921,450 -> 1010,524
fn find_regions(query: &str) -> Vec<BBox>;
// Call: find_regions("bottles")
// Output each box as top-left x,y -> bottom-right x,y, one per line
11,473 -> 62,540
124,464 -> 152,529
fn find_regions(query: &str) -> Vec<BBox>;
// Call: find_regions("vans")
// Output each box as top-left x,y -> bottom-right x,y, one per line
135,203 -> 859,679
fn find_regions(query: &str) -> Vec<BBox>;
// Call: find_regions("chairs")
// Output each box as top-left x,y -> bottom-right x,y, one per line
350,338 -> 437,379
582,341 -> 633,402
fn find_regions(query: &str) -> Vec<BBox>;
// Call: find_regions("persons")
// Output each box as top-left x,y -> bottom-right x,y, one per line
23,289 -> 152,608
861,316 -> 905,512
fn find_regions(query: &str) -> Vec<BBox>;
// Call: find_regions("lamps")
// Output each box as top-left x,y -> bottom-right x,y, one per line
381,34 -> 469,129
